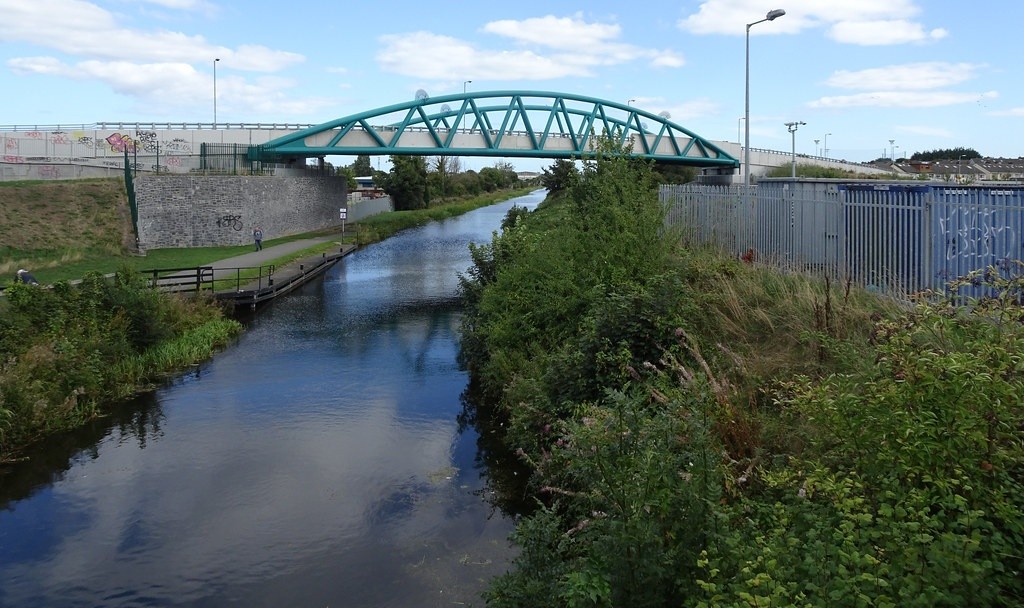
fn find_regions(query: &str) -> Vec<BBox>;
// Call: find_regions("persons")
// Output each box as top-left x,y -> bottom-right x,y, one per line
253,227 -> 262,252
14,268 -> 38,285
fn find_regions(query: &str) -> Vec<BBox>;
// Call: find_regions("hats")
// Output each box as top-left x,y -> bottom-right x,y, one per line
17,269 -> 29,275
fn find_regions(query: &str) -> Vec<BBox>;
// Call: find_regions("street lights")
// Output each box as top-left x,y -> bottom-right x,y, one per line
743,7 -> 789,195
737,117 -> 746,144
462,80 -> 473,134
892,145 -> 900,172
824,133 -> 832,159
212,57 -> 220,128
958,154 -> 966,175
627,99 -> 635,138
